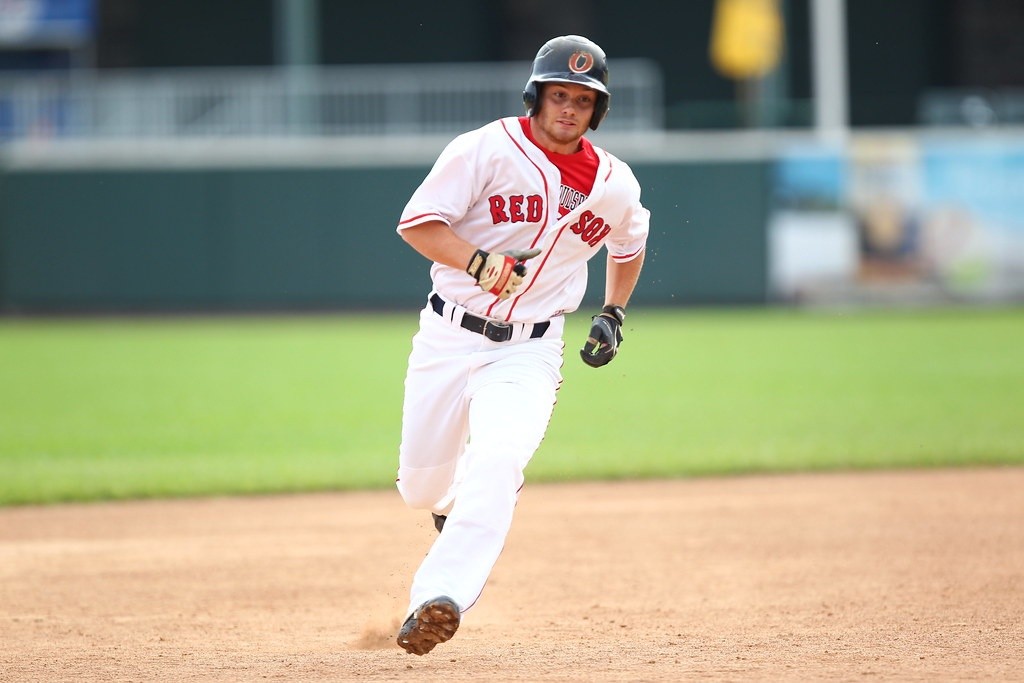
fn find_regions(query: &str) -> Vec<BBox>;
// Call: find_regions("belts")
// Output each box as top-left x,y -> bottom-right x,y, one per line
430,293 -> 551,343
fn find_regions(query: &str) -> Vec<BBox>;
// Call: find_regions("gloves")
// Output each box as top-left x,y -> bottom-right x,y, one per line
466,249 -> 543,301
580,304 -> 626,368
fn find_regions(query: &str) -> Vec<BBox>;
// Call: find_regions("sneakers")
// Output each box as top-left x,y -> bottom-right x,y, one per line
432,512 -> 445,534
396,596 -> 461,656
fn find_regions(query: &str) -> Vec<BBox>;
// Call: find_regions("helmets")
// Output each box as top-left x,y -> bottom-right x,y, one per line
524,34 -> 611,131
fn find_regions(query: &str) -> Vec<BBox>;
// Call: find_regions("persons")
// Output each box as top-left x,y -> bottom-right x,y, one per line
397,35 -> 651,656
